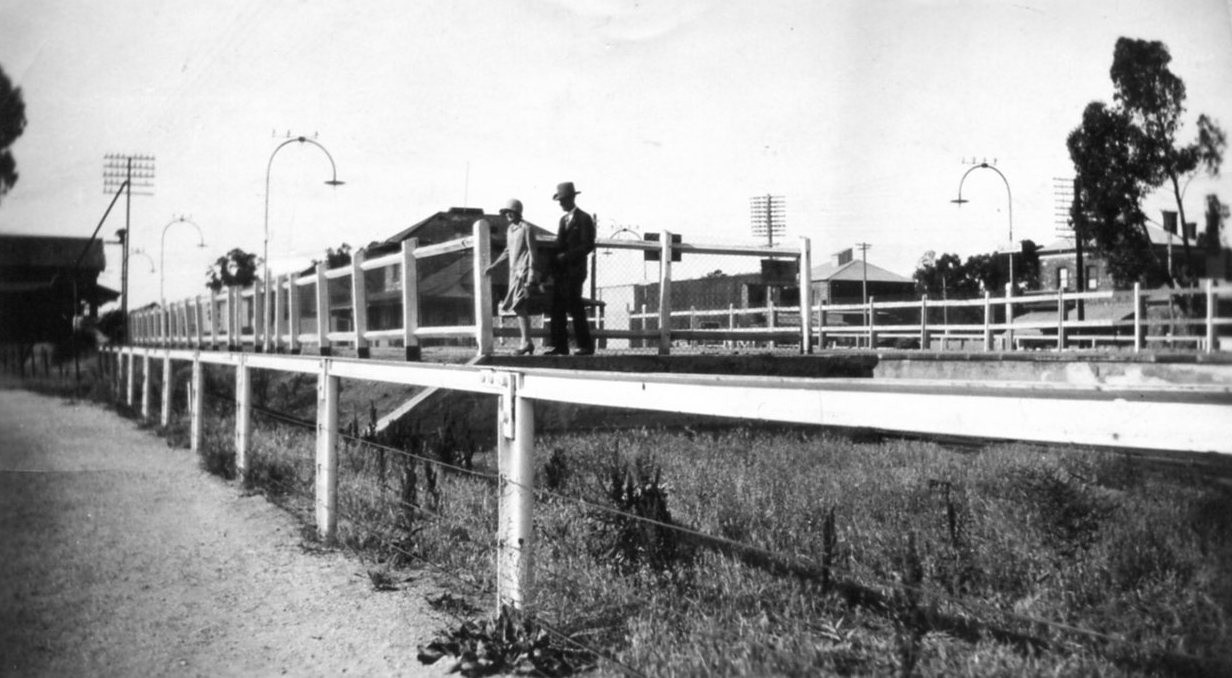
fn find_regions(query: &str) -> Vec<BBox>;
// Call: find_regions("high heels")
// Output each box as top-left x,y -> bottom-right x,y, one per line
513,343 -> 534,355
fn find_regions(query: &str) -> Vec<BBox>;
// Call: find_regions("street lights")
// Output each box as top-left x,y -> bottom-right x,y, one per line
159,212 -> 208,302
747,194 -> 787,299
103,153 -> 156,310
260,126 -> 345,280
604,224 -> 647,299
949,156 -> 1013,284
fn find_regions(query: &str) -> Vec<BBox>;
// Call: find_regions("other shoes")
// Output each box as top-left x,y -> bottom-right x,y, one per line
575,347 -> 594,355
544,348 -> 569,355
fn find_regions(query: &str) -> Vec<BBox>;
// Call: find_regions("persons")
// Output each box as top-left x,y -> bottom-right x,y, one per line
542,182 -> 596,356
480,198 -> 540,355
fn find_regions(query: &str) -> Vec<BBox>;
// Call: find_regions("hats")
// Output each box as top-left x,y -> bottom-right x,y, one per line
552,182 -> 581,200
499,199 -> 524,215
